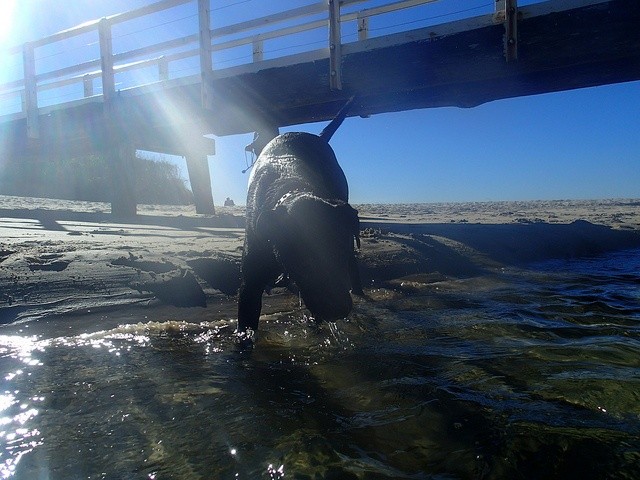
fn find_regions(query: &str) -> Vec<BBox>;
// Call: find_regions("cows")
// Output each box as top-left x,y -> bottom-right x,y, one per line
238,131 -> 362,337
